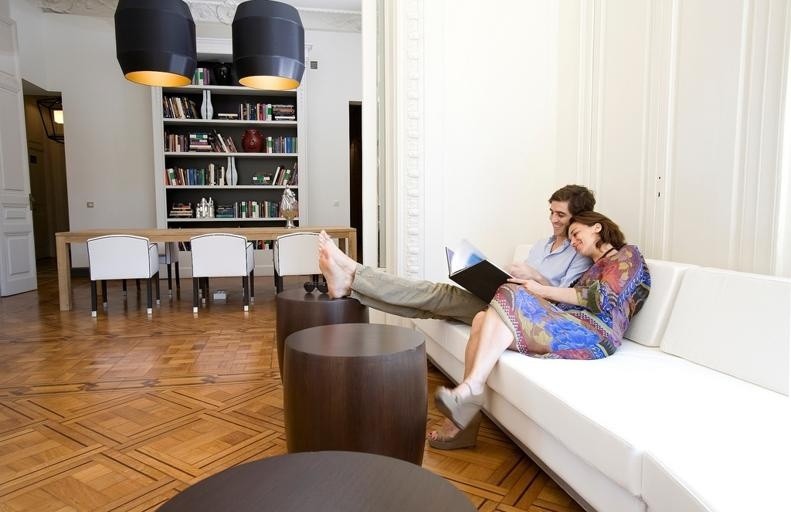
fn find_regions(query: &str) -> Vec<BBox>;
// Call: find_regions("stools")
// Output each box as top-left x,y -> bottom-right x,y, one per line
274,289 -> 370,384
283,324 -> 428,466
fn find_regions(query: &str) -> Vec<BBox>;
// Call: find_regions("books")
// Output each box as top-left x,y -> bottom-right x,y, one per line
218,102 -> 295,120
265,136 -> 298,153
165,164 -> 226,186
190,67 -> 210,85
216,200 -> 278,218
161,93 -> 199,120
251,163 -> 298,185
164,129 -> 238,152
445,245 -> 514,302
169,201 -> 194,218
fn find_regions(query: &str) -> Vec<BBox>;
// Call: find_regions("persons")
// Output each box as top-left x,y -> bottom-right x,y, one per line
428,210 -> 652,450
316,183 -> 597,325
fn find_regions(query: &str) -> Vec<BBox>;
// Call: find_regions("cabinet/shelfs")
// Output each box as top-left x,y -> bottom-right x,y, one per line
150,50 -> 309,266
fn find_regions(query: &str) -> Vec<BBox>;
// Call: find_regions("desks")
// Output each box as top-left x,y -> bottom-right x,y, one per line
54,225 -> 356,311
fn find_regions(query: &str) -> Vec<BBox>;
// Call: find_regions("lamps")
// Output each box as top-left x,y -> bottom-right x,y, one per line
36,99 -> 64,144
114,0 -> 196,86
231,0 -> 306,90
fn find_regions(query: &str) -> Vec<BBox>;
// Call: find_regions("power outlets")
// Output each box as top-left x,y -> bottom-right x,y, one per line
213,291 -> 227,299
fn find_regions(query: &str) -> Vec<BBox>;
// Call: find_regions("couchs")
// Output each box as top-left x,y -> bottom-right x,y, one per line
407,244 -> 789,511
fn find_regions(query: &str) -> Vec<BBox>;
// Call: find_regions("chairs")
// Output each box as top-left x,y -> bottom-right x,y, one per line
190,233 -> 255,313
271,232 -> 327,296
122,241 -> 180,295
85,233 -> 160,317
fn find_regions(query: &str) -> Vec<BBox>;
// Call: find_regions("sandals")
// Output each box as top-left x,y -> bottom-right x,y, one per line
434,381 -> 489,431
427,410 -> 483,450
